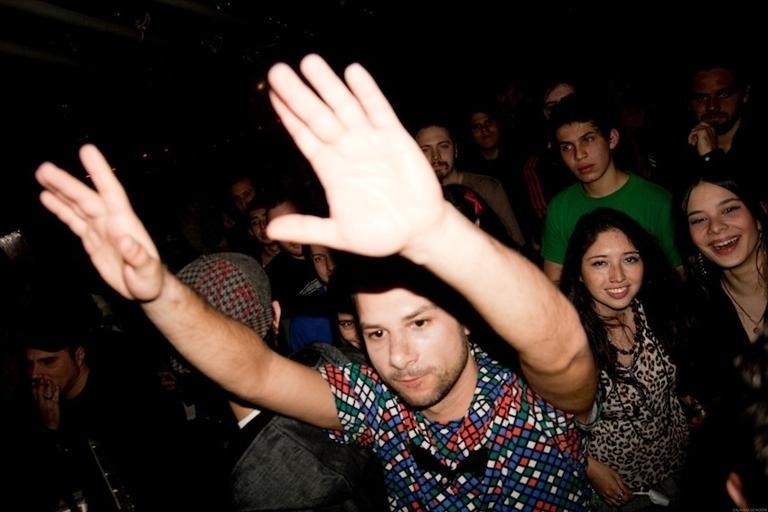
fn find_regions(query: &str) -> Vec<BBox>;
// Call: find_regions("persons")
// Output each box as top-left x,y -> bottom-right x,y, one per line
1,55 -> 767,511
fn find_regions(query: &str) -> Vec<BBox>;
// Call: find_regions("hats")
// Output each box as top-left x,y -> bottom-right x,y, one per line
171,251 -> 274,378
